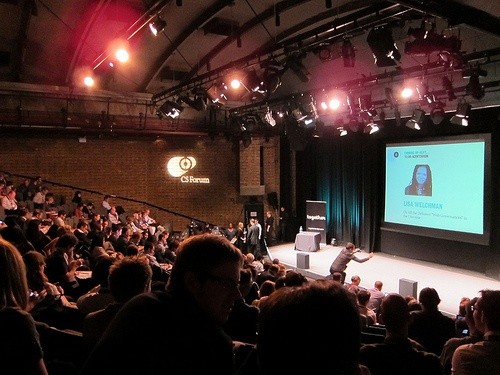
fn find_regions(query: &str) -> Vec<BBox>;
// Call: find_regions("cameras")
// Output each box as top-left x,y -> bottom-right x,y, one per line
29,292 -> 39,297
457,315 -> 462,319
462,329 -> 468,334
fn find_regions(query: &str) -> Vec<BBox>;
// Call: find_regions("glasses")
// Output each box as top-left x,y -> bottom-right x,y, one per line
470,305 -> 482,313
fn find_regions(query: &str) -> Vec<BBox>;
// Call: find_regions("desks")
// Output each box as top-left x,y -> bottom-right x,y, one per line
294,232 -> 321,252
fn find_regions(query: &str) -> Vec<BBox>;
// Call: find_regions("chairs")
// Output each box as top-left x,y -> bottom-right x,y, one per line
15,184 -> 386,375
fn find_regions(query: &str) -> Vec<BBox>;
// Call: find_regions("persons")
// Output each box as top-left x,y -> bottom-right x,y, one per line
335,273 -> 499,374
254,279 -> 375,375
323,242 -> 373,284
0,240 -> 52,375
405,165 -> 432,196
79,232 -> 247,375
0,173 -> 307,342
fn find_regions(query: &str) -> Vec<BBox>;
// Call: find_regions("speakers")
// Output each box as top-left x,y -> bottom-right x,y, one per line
297,253 -> 309,269
399,278 -> 417,299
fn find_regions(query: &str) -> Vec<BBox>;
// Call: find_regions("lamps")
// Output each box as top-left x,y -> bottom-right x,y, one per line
149,14 -> 485,147
